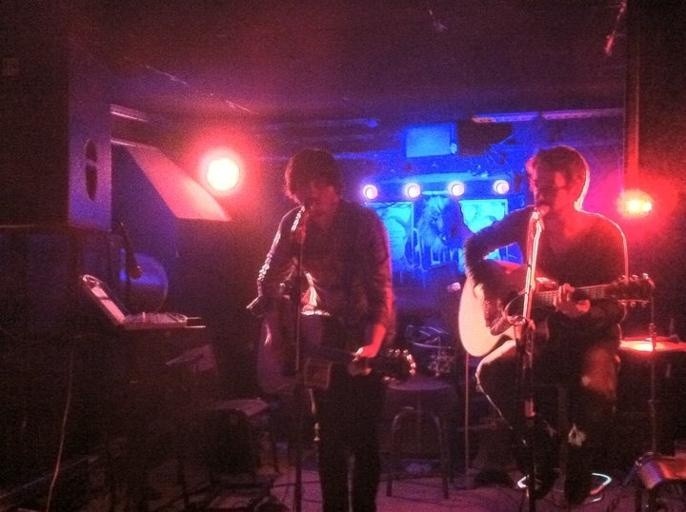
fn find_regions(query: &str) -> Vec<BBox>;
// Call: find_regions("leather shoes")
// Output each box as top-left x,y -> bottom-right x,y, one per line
532,440 -> 560,500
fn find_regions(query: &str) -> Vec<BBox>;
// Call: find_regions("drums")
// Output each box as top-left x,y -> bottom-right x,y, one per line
400,328 -> 461,380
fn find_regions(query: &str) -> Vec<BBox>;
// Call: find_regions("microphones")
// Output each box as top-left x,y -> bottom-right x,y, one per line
289,198 -> 316,239
120,219 -> 143,278
532,204 -> 550,225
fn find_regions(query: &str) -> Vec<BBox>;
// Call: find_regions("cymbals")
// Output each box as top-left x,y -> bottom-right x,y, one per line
617,333 -> 685,355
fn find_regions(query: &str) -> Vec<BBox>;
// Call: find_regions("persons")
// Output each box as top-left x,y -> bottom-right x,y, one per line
465,143 -> 629,505
247,147 -> 398,511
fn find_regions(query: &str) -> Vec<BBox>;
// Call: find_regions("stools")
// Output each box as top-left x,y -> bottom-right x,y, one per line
385,377 -> 456,501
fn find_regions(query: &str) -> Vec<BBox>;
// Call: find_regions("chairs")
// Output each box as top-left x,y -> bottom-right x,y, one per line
167,344 -> 277,511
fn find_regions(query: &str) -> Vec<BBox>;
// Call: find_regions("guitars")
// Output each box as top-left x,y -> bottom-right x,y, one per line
256,301 -> 417,396
457,261 -> 656,360
